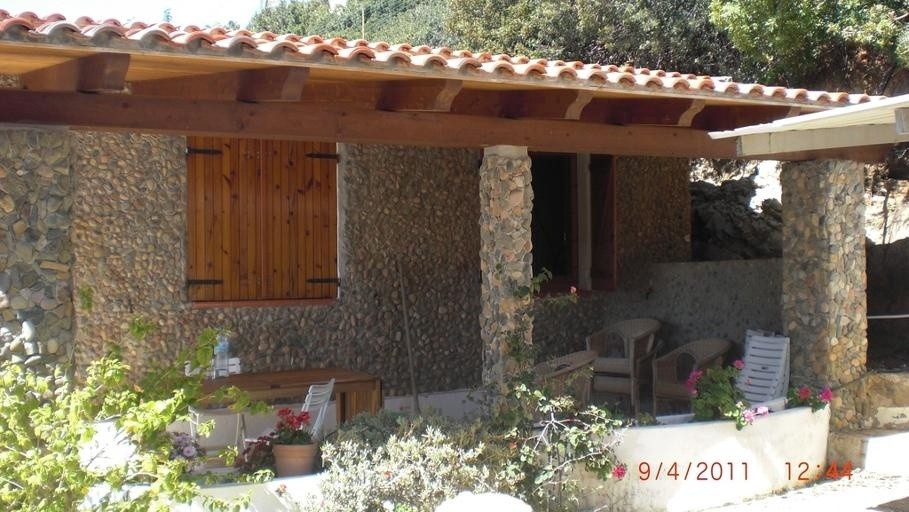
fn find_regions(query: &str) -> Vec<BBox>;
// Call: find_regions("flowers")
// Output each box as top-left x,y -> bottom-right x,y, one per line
269,407 -> 315,445
170,432 -> 208,476
788,383 -> 833,414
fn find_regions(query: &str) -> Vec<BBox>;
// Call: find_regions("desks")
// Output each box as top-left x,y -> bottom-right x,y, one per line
160,368 -> 381,447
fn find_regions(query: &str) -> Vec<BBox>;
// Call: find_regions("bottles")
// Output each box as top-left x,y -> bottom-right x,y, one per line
214,346 -> 229,380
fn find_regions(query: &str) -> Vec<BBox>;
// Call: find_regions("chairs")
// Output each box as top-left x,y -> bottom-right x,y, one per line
245,378 -> 335,462
523,316 -> 790,424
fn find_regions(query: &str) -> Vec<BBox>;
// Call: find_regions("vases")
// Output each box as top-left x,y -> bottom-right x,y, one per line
273,442 -> 320,478
